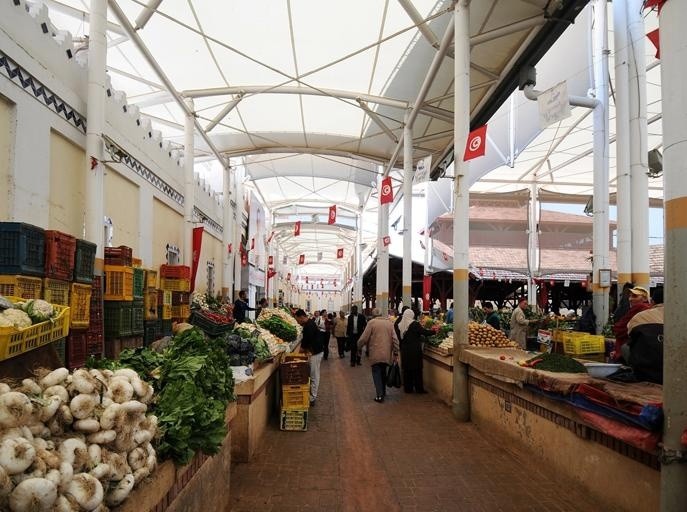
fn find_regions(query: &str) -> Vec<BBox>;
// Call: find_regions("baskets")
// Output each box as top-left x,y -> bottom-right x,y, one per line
555,329 -> 608,356
104,243 -> 192,359
189,306 -> 237,338
0,218 -> 104,376
278,350 -> 313,432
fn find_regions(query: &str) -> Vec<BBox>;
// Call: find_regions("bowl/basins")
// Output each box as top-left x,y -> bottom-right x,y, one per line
583,362 -> 623,379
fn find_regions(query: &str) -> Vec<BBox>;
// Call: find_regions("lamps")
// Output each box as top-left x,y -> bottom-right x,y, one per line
646,149 -> 664,179
101,134 -> 128,164
428,150 -> 455,182
582,195 -> 594,219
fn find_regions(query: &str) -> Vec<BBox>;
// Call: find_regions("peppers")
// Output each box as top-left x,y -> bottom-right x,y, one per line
513,360 -> 529,368
528,358 -> 542,366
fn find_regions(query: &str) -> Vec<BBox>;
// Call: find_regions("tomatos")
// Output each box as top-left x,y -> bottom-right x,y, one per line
204,311 -> 233,324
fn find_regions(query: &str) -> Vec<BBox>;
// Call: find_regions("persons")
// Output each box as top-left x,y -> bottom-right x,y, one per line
608,285 -> 652,363
507,297 -> 538,349
626,283 -> 663,384
446,302 -> 453,323
482,302 -> 501,330
233,289 -> 429,406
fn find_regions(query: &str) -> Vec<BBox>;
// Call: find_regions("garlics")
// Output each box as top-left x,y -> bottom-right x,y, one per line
1,367 -> 158,510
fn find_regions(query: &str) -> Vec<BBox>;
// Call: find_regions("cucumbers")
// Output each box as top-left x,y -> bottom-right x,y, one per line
258,316 -> 298,341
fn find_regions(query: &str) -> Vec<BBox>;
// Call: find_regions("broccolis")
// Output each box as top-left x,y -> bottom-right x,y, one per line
0,287 -> 55,328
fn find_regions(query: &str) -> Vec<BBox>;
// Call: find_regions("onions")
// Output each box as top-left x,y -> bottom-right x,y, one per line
468,323 -> 515,348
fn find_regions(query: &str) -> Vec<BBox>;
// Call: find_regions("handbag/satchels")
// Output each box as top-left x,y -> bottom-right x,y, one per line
386,359 -> 402,389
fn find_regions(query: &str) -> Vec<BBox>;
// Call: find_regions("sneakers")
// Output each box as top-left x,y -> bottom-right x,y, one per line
324,354 -> 361,370
373,390 -> 389,403
404,389 -> 428,394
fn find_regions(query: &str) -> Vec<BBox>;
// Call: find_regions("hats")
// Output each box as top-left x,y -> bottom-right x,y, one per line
623,285 -> 650,300
